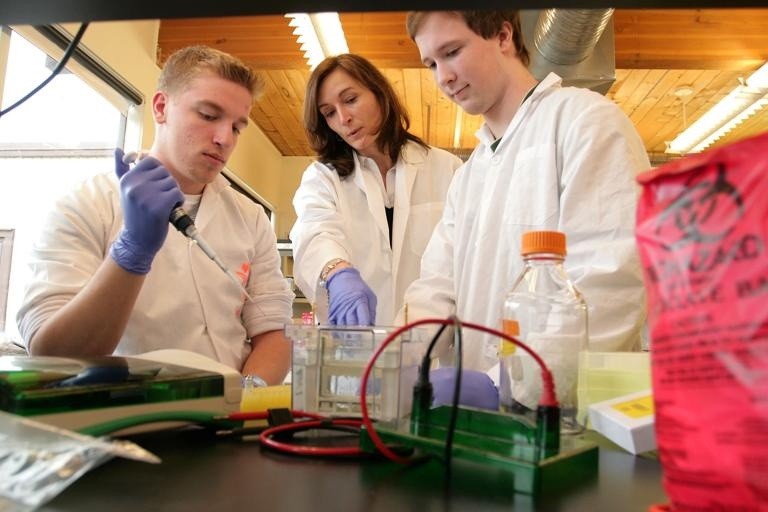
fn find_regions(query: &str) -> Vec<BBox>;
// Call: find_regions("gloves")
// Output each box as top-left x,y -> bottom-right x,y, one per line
109,146 -> 188,276
431,368 -> 501,412
324,268 -> 378,339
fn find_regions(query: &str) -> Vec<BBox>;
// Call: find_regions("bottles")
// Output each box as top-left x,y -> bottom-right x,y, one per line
499,231 -> 587,434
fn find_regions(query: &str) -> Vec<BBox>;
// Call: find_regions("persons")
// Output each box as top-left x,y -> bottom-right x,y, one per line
14,45 -> 298,388
404,7 -> 651,373
289,53 -> 464,326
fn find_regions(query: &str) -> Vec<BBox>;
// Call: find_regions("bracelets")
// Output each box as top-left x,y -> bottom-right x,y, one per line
319,259 -> 346,287
